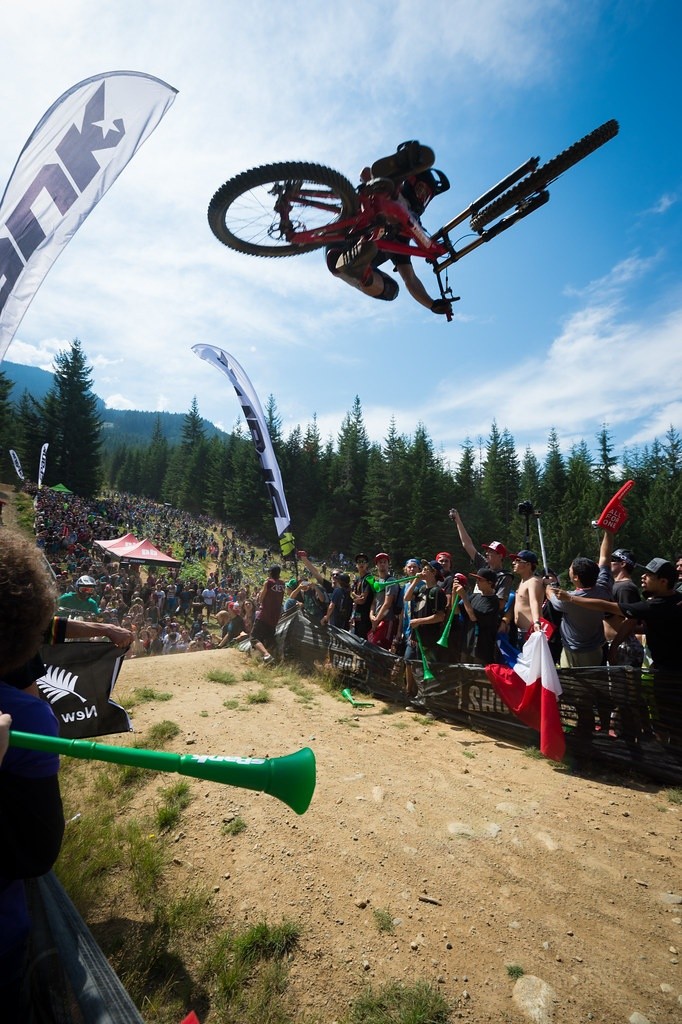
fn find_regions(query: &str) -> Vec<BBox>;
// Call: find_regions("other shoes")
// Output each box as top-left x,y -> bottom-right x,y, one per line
405,705 -> 460,724
372,144 -> 435,177
336,241 -> 378,270
262,655 -> 275,667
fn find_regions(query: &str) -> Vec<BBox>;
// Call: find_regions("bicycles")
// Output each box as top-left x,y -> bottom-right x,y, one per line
207,121 -> 621,320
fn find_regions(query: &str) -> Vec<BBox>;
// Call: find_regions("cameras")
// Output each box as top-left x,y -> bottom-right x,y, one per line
518,501 -> 534,515
551,581 -> 561,589
533,509 -> 542,517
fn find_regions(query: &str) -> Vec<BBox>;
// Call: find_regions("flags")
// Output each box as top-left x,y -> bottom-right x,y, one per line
36,641 -> 133,740
0,74 -> 177,359
485,632 -> 566,761
194,346 -> 296,563
9,443 -> 48,487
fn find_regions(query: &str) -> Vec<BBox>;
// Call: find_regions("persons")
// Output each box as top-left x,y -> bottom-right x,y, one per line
399,508 -> 682,761
332,142 -> 454,314
0,528 -> 135,1024
249,565 -> 285,669
22,478 -> 400,692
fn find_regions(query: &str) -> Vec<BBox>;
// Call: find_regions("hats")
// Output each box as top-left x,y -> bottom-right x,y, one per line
285,579 -> 297,590
436,552 -> 452,562
270,564 -> 281,579
509,549 -> 539,565
455,573 -> 467,587
405,559 -> 421,570
468,568 -> 497,583
482,540 -> 507,558
336,573 -> 350,584
375,552 -> 390,564
610,548 -> 634,568
635,557 -> 678,584
355,552 -> 368,561
421,559 -> 445,582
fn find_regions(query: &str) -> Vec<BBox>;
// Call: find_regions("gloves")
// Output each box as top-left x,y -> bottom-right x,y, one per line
429,295 -> 461,315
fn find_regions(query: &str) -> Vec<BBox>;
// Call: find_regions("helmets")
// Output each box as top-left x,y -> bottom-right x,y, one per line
75,575 -> 96,600
400,168 -> 450,216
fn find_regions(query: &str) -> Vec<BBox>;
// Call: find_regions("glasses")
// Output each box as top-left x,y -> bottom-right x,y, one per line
515,558 -> 527,563
415,181 -> 432,207
78,586 -> 94,594
356,559 -> 368,563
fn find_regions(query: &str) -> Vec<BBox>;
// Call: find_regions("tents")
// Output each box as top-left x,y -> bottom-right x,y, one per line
49,483 -> 73,494
106,538 -> 182,573
93,533 -> 140,560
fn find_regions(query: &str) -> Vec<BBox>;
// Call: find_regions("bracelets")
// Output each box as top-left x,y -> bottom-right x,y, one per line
534,621 -> 541,625
